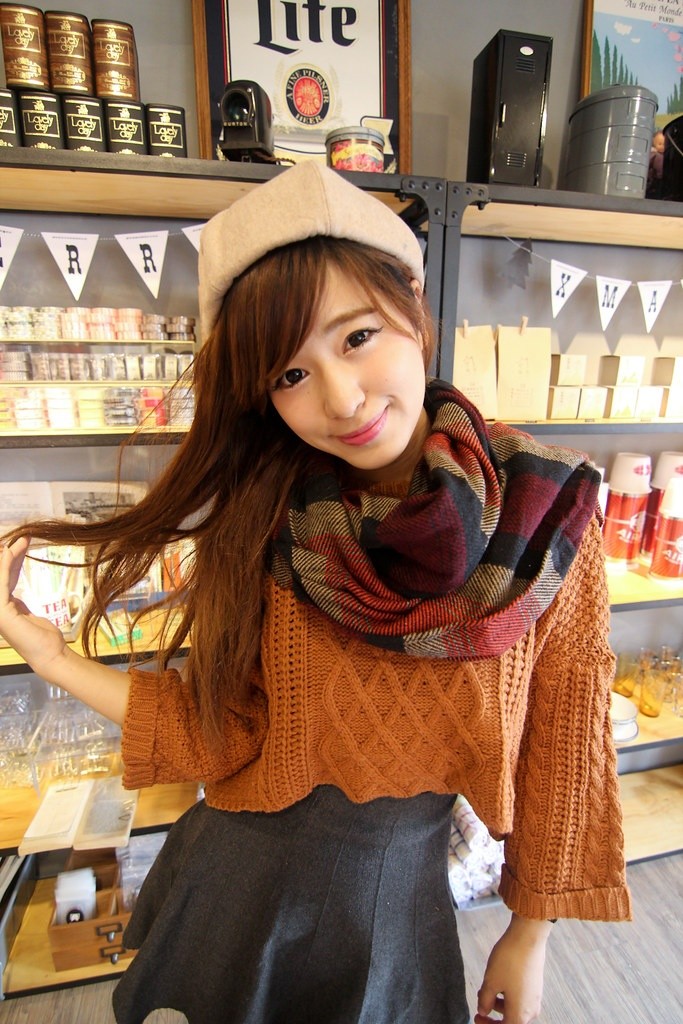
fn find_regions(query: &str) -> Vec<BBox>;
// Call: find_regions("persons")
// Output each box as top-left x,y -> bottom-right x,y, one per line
0,156 -> 633,1023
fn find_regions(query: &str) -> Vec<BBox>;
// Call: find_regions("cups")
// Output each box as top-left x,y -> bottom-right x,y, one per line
324,126 -> 385,174
611,645 -> 683,717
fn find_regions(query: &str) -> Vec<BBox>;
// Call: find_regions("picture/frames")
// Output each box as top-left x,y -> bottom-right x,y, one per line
580,0 -> 683,202
192,0 -> 413,175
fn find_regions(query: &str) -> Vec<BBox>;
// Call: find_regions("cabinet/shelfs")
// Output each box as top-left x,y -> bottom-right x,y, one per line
439,174 -> 683,909
0,147 -> 443,1002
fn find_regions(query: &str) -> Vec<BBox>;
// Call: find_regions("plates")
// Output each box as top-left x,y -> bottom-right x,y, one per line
610,692 -> 638,730
611,722 -> 639,743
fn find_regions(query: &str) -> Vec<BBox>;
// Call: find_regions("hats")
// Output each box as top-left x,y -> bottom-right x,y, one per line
198,159 -> 425,346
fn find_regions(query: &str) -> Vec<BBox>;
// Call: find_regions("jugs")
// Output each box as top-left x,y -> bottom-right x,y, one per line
601,451 -> 683,587
